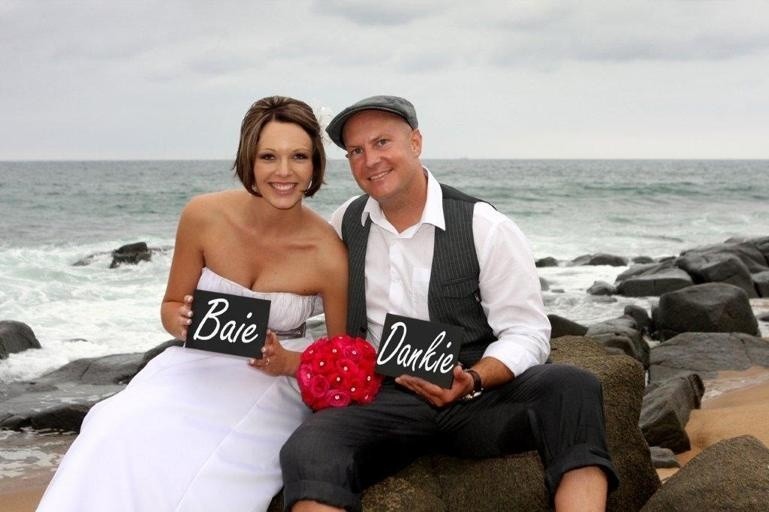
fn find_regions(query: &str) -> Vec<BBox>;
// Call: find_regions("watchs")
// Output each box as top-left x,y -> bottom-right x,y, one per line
460,368 -> 484,403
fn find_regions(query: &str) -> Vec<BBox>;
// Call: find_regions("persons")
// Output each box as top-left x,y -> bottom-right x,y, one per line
276,92 -> 620,512
36,93 -> 353,510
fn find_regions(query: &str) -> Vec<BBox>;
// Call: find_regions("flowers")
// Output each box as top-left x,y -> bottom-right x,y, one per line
296,335 -> 382,413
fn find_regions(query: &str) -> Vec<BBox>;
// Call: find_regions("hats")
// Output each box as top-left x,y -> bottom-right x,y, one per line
325,94 -> 420,151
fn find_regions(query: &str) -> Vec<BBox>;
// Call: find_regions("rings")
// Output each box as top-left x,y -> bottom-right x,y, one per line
266,357 -> 270,365
